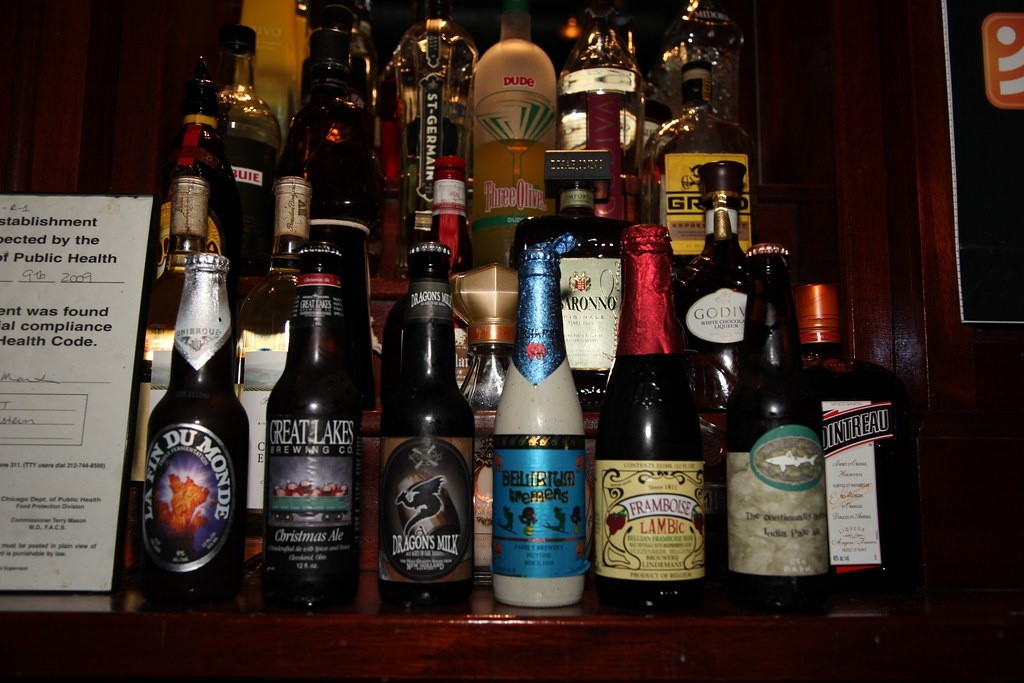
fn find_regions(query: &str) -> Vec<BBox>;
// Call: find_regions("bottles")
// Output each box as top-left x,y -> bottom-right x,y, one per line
378,241 -> 473,614
156,2 -> 758,273
780,281 -> 920,607
224,175 -> 335,570
119,163 -> 248,575
260,247 -> 361,617
589,223 -> 709,619
512,146 -> 636,408
711,242 -> 834,613
669,162 -> 793,478
490,248 -> 593,608
131,253 -> 249,615
439,263 -> 536,578
